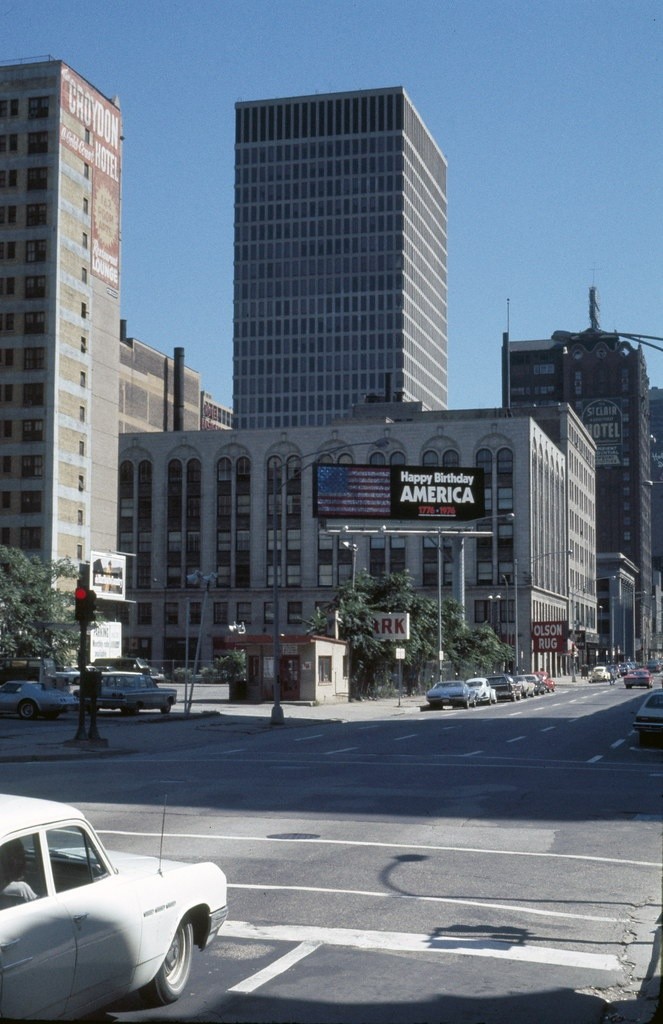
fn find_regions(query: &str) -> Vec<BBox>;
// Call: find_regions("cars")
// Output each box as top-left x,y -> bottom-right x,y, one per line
623,668 -> 655,689
0,678 -> 80,720
465,677 -> 498,706
51,662 -> 100,697
632,689 -> 663,746
97,667 -> 179,716
425,680 -> 478,711
485,674 -> 524,701
1,787 -> 232,1021
587,658 -> 663,683
512,670 -> 556,698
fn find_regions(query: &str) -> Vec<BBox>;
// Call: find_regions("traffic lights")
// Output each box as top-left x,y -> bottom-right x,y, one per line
87,589 -> 97,621
73,587 -> 88,622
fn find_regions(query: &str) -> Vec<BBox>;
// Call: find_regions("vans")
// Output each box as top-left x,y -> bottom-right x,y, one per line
93,656 -> 166,685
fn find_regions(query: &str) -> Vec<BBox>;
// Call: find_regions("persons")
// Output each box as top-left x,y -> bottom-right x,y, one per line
0,839 -> 38,900
610,666 -> 615,685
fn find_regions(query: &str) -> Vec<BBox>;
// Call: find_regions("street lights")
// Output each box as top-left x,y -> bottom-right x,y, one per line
623,595 -> 656,662
342,541 -> 358,587
265,439 -> 392,722
437,511 -> 516,683
611,590 -> 648,663
514,549 -> 573,676
570,575 -> 618,683
